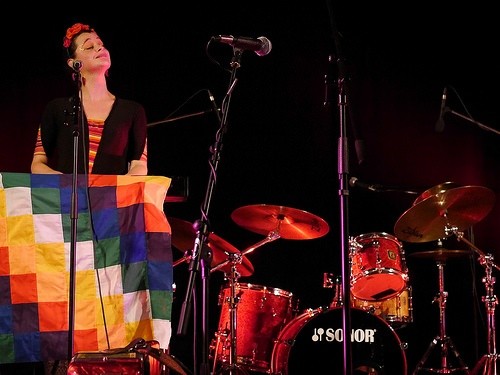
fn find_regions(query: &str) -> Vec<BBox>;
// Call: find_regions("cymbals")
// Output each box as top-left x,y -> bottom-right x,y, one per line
231,204 -> 329,240
165,195 -> 188,203
394,186 -> 496,243
409,248 -> 475,261
166,216 -> 255,277
413,182 -> 458,206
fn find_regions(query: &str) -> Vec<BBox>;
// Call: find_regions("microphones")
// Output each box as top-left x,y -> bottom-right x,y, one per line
71,59 -> 84,71
436,88 -> 447,130
349,177 -> 375,191
213,36 -> 272,56
208,90 -> 223,122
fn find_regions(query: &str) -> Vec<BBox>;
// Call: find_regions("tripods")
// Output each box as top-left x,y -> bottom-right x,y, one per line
209,217 -> 282,375
412,210 -> 500,375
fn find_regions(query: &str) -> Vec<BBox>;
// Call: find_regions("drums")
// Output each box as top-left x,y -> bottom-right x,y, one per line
349,232 -> 409,302
332,276 -> 414,327
209,283 -> 299,373
270,305 -> 407,375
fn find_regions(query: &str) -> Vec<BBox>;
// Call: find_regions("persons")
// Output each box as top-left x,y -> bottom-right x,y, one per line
31,23 -> 148,176
